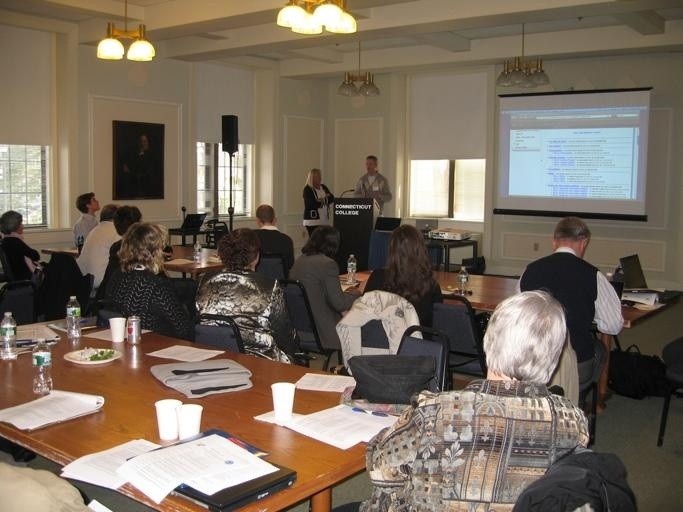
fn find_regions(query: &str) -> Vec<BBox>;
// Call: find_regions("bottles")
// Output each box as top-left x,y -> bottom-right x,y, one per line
31,338 -> 53,395
0,312 -> 17,360
194,241 -> 202,263
77,232 -> 84,254
127,345 -> 143,369
347,254 -> 357,283
456,266 -> 469,298
66,296 -> 81,344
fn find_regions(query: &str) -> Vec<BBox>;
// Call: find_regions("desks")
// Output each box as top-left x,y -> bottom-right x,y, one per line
165,246 -> 226,279
41,247 -> 79,258
0,320 -> 399,512
423,236 -> 478,273
168,228 -> 214,245
338,269 -> 674,414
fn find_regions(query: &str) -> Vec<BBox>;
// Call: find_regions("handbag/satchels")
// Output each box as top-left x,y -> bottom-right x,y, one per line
607,344 -> 653,401
348,354 -> 438,405
646,353 -> 679,398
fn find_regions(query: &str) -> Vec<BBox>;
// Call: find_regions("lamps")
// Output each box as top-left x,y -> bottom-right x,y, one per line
96,0 -> 155,61
336,39 -> 380,97
277,0 -> 357,35
495,23 -> 549,87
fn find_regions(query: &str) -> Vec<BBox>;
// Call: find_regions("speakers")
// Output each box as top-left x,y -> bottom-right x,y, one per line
222,115 -> 238,153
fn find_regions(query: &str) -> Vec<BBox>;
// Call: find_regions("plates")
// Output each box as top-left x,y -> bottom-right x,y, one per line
62,348 -> 122,368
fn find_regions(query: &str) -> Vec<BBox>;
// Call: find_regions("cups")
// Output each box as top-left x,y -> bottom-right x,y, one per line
155,399 -> 182,442
271,382 -> 296,418
109,318 -> 126,343
175,404 -> 204,441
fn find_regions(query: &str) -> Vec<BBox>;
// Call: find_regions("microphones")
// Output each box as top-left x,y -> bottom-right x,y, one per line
362,184 -> 368,198
340,189 -> 355,198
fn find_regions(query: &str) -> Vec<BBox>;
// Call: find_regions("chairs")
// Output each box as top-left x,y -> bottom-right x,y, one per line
213,222 -> 229,248
433,295 -> 488,378
397,325 -> 453,391
191,315 -> 245,353
257,255 -> 287,280
581,383 -> 596,445
0,280 -> 38,325
656,335 -> 683,446
280,278 -> 342,372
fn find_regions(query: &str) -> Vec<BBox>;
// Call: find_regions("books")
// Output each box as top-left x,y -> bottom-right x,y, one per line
0,326 -> 60,346
0,389 -> 105,432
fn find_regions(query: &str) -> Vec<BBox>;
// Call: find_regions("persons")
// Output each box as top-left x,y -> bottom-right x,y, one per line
73,192 -> 100,246
114,222 -> 193,340
290,226 -> 361,367
94,206 -> 142,299
362,224 -> 443,330
0,211 -> 40,280
194,227 -> 307,366
76,204 -> 123,293
354,156 -> 393,217
248,204 -> 295,276
517,218 -> 624,388
302,168 -> 334,232
122,132 -> 155,194
359,288 -> 590,512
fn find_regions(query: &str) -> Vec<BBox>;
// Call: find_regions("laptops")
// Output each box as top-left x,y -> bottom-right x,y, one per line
608,282 -> 624,300
125,432 -> 298,511
168,214 -> 207,233
619,254 -> 682,301
416,219 -> 438,232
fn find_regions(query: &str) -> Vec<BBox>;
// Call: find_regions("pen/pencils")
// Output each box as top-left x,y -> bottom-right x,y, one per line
353,407 -> 389,418
